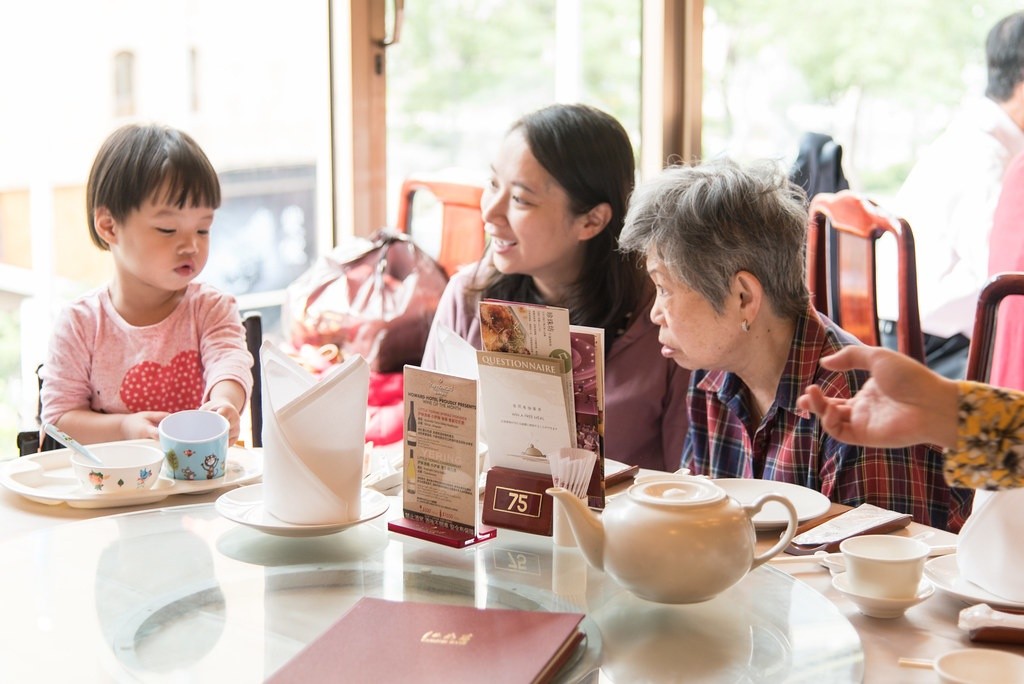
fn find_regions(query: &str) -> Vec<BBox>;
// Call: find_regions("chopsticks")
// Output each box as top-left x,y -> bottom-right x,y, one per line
899,658 -> 934,666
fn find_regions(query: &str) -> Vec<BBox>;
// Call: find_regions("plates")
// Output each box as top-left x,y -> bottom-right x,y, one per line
214,483 -> 390,537
832,570 -> 934,618
923,554 -> 1024,610
710,478 -> 831,531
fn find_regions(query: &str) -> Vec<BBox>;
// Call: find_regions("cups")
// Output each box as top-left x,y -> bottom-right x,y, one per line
158,410 -> 229,479
553,495 -> 588,546
935,648 -> 1024,684
634,474 -> 713,485
839,535 -> 930,599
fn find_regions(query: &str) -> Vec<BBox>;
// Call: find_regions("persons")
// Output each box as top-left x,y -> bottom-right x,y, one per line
419,105 -> 1024,536
39,119 -> 254,446
871,9 -> 1023,380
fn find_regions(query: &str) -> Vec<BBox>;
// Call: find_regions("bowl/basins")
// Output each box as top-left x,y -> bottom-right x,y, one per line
71,445 -> 166,492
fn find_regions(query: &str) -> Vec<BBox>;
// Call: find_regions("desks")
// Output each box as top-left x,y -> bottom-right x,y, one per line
0,444 -> 1024,684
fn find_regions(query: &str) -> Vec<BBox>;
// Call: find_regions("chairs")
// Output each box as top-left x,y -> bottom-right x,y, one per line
964,270 -> 1024,384
805,188 -> 925,368
395,177 -> 490,279
18,310 -> 265,457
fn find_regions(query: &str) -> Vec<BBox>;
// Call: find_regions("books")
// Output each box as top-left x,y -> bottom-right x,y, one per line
267,595 -> 586,684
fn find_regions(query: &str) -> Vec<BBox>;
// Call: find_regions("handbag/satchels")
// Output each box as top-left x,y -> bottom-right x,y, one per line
280,227 -> 452,372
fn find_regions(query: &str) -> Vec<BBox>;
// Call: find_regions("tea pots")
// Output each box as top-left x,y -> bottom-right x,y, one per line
545,480 -> 797,604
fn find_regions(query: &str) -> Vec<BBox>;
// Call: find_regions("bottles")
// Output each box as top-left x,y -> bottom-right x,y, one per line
407,401 -> 416,446
407,449 -> 416,494
